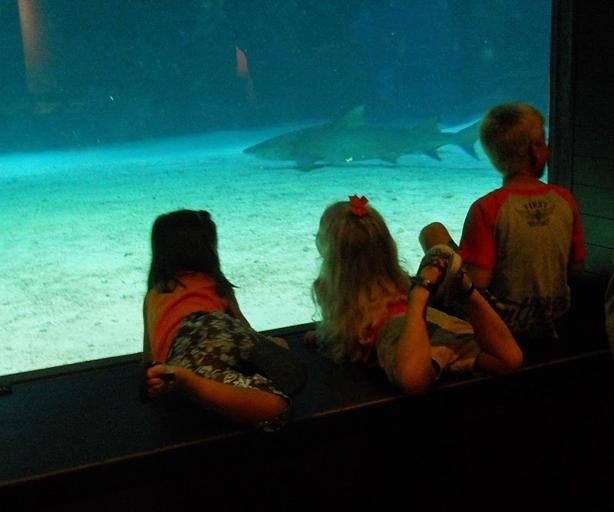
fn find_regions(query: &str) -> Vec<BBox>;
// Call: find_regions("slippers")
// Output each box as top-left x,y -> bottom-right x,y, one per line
136,359 -> 175,398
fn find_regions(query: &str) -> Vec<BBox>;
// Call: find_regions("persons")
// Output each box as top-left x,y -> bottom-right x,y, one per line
302,194 -> 526,399
143,208 -> 308,434
419,103 -> 586,335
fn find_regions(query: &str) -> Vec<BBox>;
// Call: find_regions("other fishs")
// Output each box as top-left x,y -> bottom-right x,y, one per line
242,103 -> 483,172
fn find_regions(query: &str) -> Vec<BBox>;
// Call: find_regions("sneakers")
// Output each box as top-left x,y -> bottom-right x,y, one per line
408,243 -> 463,307
